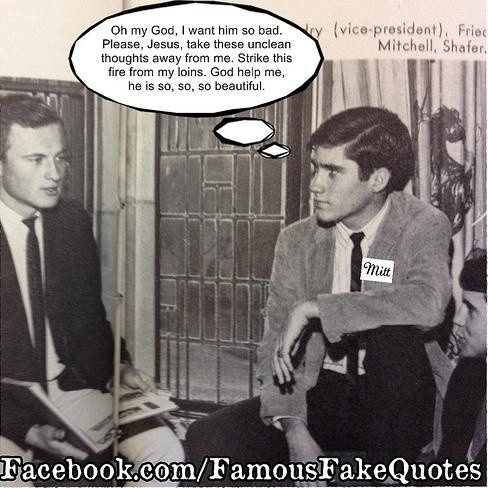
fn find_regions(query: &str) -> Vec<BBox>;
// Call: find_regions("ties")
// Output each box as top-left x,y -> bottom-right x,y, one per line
347,233 -> 364,375
22,217 -> 48,396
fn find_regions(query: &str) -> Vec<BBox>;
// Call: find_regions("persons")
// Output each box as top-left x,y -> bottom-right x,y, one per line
416,253 -> 486,486
179,105 -> 455,487
0,92 -> 186,487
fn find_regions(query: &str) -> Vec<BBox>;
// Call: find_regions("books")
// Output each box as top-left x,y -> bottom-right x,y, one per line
1,375 -> 179,458
